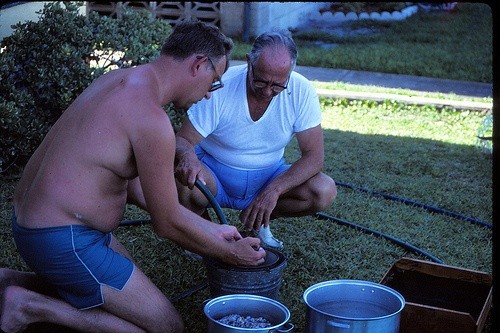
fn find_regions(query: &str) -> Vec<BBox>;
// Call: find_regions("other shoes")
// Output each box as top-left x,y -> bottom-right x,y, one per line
254,224 -> 287,251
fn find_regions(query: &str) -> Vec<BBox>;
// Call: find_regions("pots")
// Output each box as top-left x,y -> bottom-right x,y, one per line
199,293 -> 294,333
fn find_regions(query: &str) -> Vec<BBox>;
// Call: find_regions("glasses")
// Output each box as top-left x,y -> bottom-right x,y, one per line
196,54 -> 224,92
249,62 -> 290,92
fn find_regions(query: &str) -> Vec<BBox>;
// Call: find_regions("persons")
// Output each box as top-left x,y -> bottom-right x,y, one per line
0,19 -> 267,333
168,30 -> 338,264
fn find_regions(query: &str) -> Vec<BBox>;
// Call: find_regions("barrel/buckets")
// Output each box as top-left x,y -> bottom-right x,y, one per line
203,242 -> 286,302
302,280 -> 405,333
